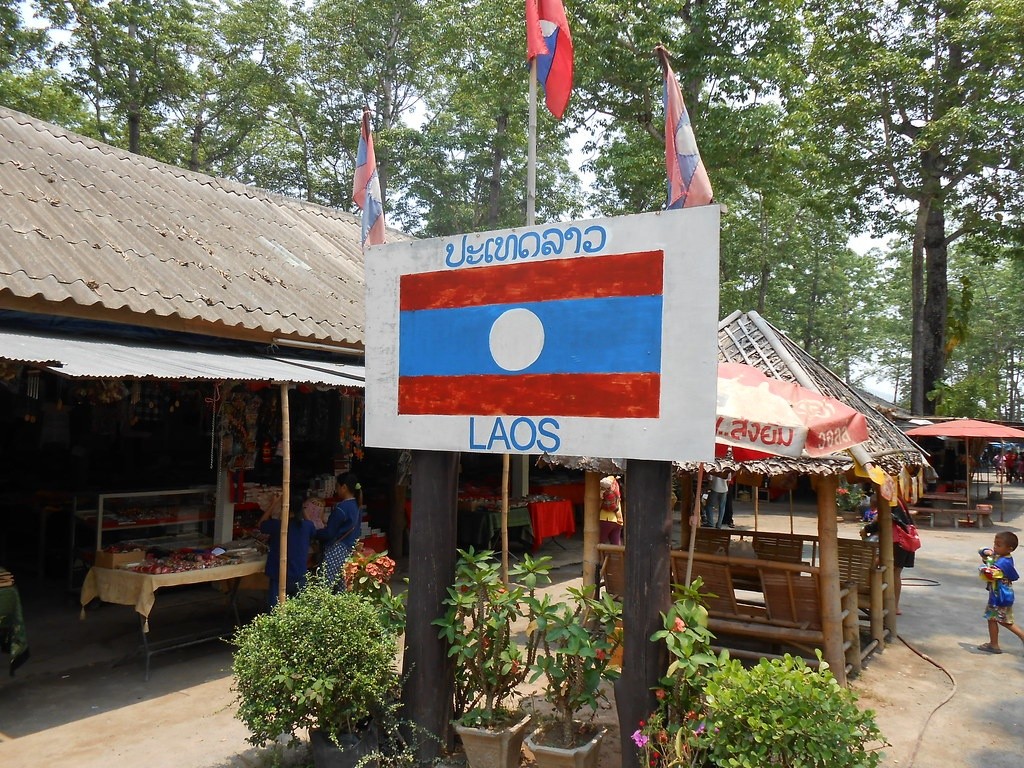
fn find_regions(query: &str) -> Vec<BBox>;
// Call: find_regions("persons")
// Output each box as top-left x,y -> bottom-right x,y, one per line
925,464 -> 939,507
859,483 -> 922,614
993,450 -> 1024,487
692,471 -> 737,529
316,473 -> 363,593
597,474 -> 622,545
256,490 -> 316,606
978,532 -> 1024,654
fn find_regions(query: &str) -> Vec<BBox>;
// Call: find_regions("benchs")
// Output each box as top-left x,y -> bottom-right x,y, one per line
906,506 -> 991,531
598,525 -> 887,681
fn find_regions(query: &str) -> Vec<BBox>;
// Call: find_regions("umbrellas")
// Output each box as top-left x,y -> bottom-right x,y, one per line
680,363 -> 870,605
904,418 -> 1024,523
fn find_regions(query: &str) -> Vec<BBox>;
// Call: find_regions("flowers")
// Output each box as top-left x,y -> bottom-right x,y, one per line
836,484 -> 867,511
430,547 -> 717,768
339,541 -> 411,638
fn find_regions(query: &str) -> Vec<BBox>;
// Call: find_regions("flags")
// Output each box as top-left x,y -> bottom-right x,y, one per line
352,110 -> 388,254
662,60 -> 716,212
525,0 -> 574,119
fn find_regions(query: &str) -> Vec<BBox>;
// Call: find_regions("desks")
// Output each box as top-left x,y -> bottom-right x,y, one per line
80,545 -> 270,681
455,483 -> 585,566
307,534 -> 387,569
730,558 -> 812,583
920,493 -> 967,526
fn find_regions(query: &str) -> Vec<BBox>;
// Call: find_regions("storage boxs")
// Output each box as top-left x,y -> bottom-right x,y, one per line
94,549 -> 145,569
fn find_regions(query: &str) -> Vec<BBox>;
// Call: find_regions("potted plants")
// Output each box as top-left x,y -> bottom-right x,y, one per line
218,537 -> 465,768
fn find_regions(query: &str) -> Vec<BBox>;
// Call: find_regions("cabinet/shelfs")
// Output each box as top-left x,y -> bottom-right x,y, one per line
68,484 -> 272,610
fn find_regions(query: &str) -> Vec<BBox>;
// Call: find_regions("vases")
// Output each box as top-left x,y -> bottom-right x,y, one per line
842,511 -> 858,522
522,719 -> 609,768
453,709 -> 532,768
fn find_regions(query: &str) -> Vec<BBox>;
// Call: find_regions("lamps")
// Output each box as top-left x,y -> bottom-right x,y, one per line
272,337 -> 364,355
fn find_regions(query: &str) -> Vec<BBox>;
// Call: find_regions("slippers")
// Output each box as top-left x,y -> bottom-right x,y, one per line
978,642 -> 1002,654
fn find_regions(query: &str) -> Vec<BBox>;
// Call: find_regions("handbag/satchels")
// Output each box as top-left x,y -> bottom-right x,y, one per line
311,551 -> 324,569
892,521 -> 921,552
998,584 -> 1014,606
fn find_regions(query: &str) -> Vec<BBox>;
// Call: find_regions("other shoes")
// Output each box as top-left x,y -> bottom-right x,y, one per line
728,522 -> 734,528
701,523 -> 713,527
